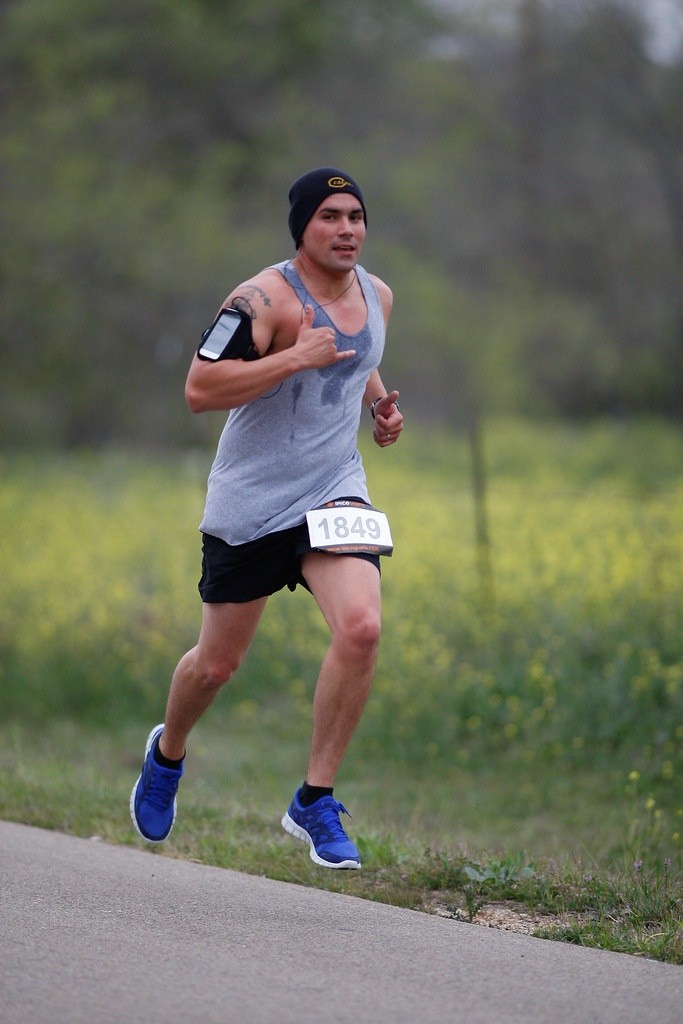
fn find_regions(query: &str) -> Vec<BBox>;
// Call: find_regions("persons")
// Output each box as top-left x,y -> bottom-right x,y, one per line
130,169 -> 404,868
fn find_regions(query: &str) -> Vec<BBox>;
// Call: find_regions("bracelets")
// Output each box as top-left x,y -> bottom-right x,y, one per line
370,397 -> 400,420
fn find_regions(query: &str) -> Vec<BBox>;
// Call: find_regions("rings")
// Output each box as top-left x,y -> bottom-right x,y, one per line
386,434 -> 390,440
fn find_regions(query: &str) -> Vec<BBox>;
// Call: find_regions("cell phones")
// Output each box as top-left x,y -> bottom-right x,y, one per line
197,310 -> 245,362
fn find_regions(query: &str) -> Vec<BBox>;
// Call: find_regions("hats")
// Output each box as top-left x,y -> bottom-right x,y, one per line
289,169 -> 367,248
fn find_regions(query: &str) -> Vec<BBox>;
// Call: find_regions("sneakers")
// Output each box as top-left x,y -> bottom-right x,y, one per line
128,724 -> 184,842
281,785 -> 362,870
